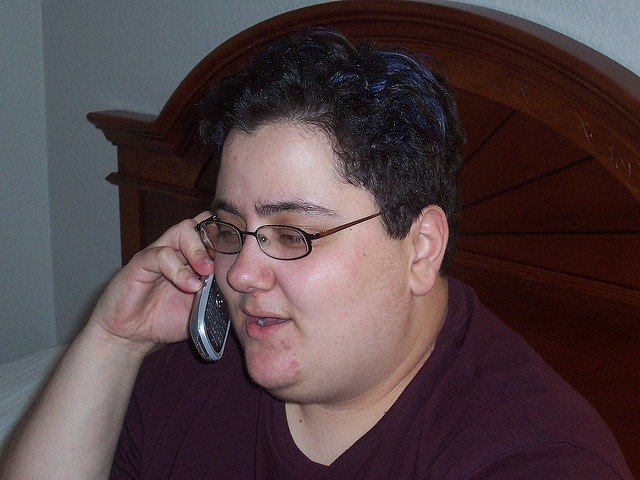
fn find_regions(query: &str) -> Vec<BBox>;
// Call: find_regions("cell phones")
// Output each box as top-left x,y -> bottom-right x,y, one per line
188,274 -> 231,362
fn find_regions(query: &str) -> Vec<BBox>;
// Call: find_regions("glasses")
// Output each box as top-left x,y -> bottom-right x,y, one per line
193,196 -> 426,261
